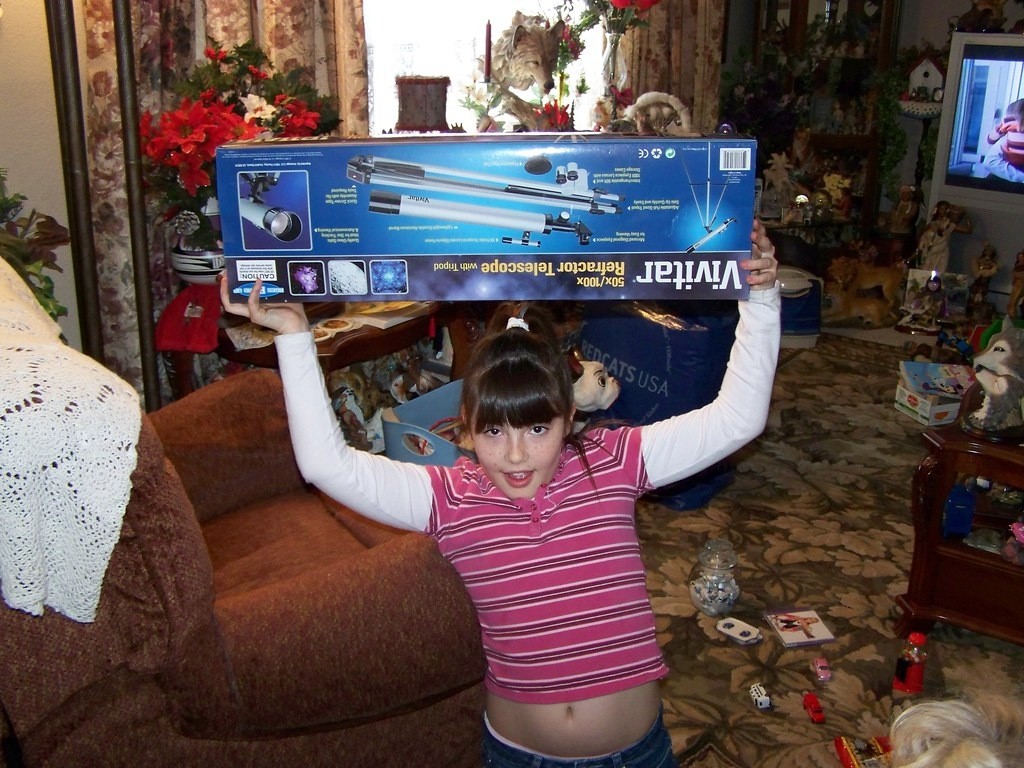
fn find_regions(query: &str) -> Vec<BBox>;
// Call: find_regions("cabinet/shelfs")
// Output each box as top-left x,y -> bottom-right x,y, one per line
889,422 -> 1024,645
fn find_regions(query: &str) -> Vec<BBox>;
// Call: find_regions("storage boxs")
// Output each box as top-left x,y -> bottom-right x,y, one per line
213,132 -> 760,305
892,386 -> 960,428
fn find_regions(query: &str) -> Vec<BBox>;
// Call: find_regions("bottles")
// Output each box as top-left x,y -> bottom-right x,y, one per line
893,632 -> 929,694
687,538 -> 742,618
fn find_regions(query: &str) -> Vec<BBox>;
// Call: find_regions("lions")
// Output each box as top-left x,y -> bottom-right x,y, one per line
826,254 -> 907,308
821,280 -> 905,328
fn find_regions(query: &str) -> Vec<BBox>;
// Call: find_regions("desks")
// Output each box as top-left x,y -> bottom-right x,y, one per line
158,301 -> 488,404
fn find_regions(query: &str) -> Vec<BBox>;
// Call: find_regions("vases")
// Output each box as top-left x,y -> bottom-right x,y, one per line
599,34 -> 628,94
169,211 -> 228,285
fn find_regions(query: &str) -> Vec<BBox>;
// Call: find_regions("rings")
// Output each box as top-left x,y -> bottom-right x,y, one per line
216,274 -> 223,284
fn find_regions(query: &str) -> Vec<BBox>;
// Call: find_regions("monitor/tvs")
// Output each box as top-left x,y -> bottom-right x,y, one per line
945,44 -> 1024,195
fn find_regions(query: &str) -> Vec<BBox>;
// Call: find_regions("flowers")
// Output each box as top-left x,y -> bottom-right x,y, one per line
532,0 -> 659,134
134,41 -> 344,253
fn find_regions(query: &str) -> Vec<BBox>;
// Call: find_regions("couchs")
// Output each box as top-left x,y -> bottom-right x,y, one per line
0,254 -> 487,768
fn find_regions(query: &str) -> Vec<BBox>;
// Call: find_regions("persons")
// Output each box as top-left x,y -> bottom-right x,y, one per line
216,220 -> 783,768
1007,252 -> 1024,318
970,245 -> 998,302
832,187 -> 852,221
917,200 -> 971,271
773,613 -> 819,638
890,186 -> 918,233
